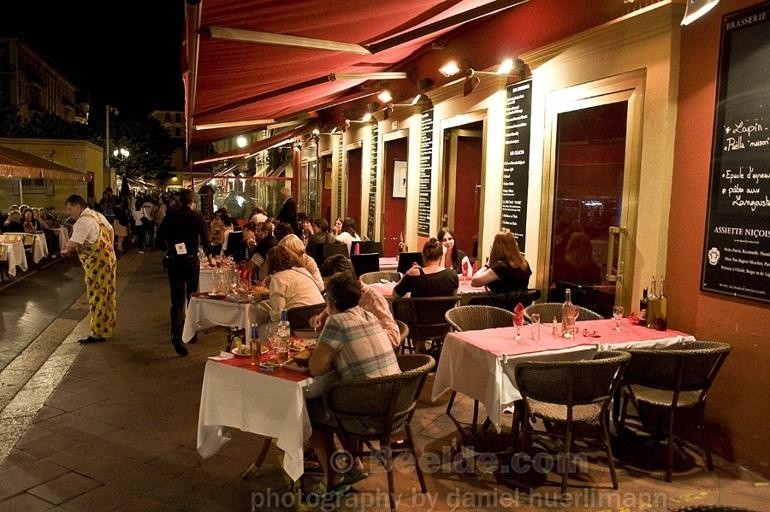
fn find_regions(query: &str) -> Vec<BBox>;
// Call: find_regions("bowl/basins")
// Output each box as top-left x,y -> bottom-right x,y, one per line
292,348 -> 312,366
294,329 -> 321,337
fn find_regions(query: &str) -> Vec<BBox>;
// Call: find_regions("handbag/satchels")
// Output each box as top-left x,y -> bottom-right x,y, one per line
141,216 -> 151,228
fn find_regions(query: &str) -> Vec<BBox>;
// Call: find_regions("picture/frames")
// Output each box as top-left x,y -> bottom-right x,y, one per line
391,158 -> 407,200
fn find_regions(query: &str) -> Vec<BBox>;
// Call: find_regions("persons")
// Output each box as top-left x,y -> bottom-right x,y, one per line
548,201 -> 620,318
1,182 -> 535,503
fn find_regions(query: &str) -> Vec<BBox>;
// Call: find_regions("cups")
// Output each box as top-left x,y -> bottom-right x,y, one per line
353,243 -> 359,255
198,247 -> 269,303
531,313 -> 541,340
572,306 -> 579,321
59,225 -> 63,228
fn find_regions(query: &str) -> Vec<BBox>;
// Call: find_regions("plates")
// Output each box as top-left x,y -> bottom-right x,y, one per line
232,344 -> 269,356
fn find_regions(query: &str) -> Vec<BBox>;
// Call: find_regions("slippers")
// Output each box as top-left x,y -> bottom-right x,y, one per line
313,472 -> 369,501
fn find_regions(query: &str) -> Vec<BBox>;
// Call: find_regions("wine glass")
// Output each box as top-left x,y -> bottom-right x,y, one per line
513,315 -> 524,340
613,306 -> 624,332
461,265 -> 468,286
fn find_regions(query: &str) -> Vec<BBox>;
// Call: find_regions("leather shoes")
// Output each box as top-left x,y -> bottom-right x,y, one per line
78,336 -> 106,344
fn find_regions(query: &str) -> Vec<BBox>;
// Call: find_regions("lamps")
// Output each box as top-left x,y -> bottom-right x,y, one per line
293,58 -> 527,153
679,0 -> 720,27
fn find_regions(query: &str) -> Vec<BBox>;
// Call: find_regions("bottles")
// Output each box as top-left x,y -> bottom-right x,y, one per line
265,307 -> 292,371
399,239 -> 408,252
249,324 -> 262,366
563,288 -> 575,340
300,230 -> 306,245
639,278 -> 667,330
552,313 -> 558,335
25,221 -> 43,233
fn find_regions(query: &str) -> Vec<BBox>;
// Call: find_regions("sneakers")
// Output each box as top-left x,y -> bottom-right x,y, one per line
171,336 -> 189,356
138,251 -> 144,254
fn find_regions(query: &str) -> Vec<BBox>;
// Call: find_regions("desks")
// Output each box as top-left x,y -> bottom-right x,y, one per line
0,227 -> 68,274
